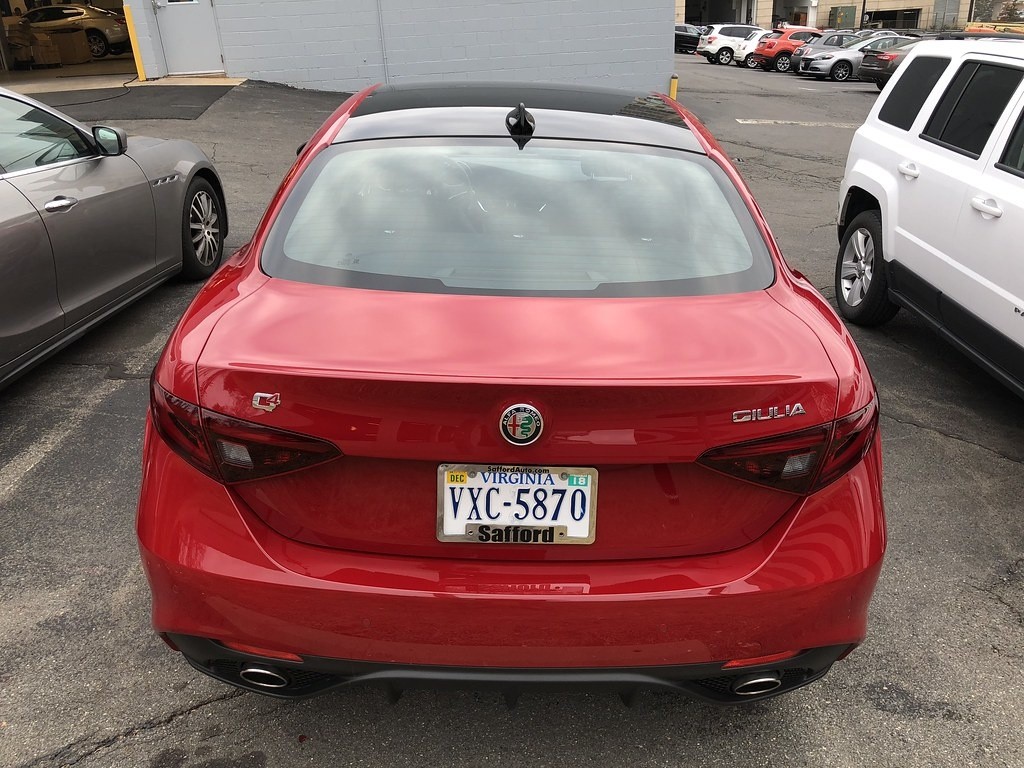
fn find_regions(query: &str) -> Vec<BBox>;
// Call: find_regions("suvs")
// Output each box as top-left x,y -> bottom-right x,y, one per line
790,32 -> 863,76
696,22 -> 766,66
833,32 -> 1024,404
753,27 -> 825,72
675,23 -> 704,54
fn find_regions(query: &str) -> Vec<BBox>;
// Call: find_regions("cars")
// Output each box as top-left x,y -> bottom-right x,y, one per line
133,77 -> 890,712
799,34 -> 919,83
784,24 -> 899,37
733,30 -> 774,68
858,39 -> 937,92
0,86 -> 232,390
14,3 -> 132,59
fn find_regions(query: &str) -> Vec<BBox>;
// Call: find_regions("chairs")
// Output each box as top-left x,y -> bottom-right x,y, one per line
356,154 -> 487,241
